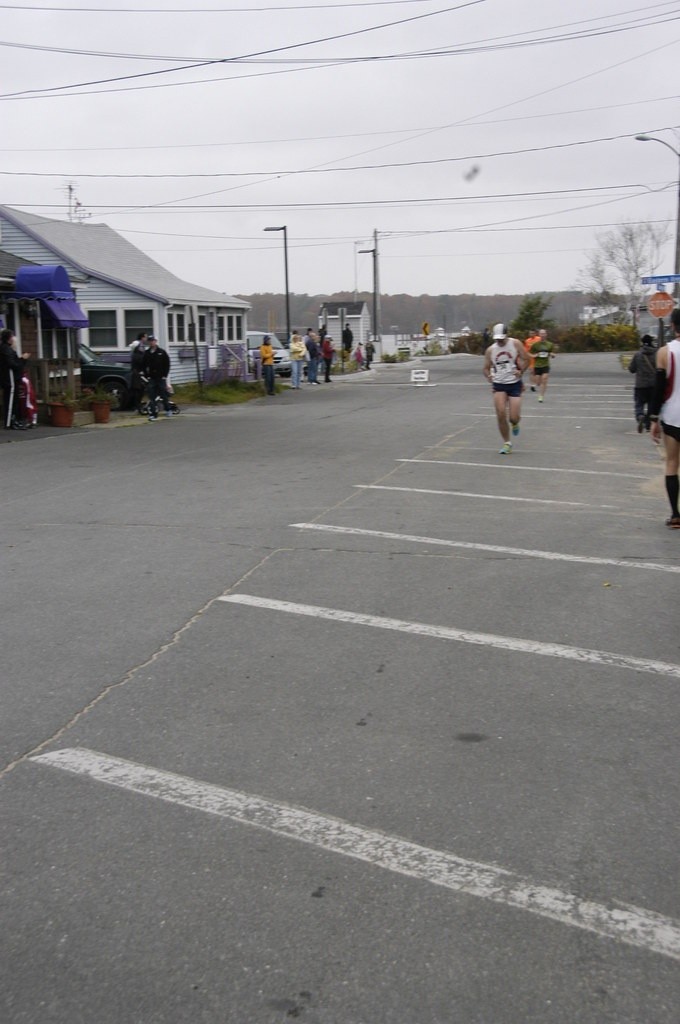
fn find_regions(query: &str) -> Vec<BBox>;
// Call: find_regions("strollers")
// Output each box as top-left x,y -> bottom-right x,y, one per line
137,373 -> 181,416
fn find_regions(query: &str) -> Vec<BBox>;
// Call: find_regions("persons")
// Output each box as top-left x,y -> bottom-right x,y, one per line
288,324 -> 338,389
342,323 -> 353,352
524,329 -> 556,402
482,324 -> 531,454
0,330 -> 31,430
260,335 -> 278,396
650,308 -> 680,529
481,327 -> 489,355
130,333 -> 174,421
351,339 -> 375,372
628,334 -> 657,433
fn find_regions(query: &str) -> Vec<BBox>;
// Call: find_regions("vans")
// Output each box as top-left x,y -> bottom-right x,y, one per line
246,330 -> 293,379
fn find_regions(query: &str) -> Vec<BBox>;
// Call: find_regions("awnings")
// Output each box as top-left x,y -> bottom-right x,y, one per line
0,264 -> 90,328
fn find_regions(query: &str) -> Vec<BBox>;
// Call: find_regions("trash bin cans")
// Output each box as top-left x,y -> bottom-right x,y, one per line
397,347 -> 410,358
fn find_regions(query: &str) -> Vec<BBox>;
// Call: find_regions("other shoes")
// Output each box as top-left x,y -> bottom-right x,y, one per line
309,380 -> 321,385
365,364 -> 370,370
666,517 -> 680,528
267,391 -> 275,395
147,415 -> 158,420
167,409 -> 172,417
13,422 -> 27,430
292,385 -> 296,389
538,395 -> 544,402
638,414 -> 645,433
324,379 -> 332,383
645,421 -> 650,430
531,385 -> 537,392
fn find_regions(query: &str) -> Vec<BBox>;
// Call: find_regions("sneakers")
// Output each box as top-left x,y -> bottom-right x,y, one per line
511,423 -> 520,436
499,442 -> 513,454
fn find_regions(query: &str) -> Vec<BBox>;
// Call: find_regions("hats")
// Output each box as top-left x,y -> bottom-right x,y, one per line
492,323 -> 508,340
325,334 -> 332,339
147,335 -> 156,341
641,335 -> 653,344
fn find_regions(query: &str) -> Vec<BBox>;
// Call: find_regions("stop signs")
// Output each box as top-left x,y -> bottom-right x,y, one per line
648,292 -> 674,319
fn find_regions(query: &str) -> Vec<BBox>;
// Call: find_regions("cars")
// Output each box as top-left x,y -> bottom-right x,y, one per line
76,343 -> 135,414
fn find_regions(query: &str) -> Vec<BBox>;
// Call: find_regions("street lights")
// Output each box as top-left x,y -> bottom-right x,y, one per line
635,135 -> 680,300
358,249 -> 380,342
263,225 -> 291,342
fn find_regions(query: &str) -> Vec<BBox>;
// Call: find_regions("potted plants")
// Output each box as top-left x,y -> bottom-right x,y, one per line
47,389 -> 80,427
86,383 -> 117,423
